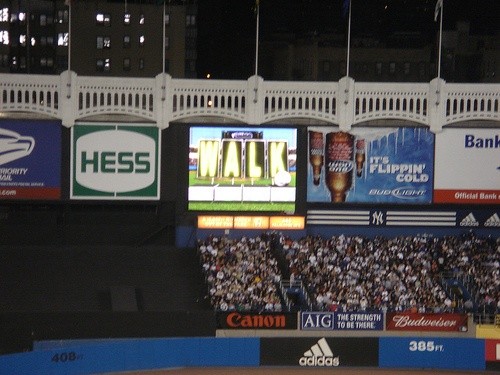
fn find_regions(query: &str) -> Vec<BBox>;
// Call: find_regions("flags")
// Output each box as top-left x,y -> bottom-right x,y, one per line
343,0 -> 350,18
64,0 -> 70,6
248,1 -> 257,16
434,0 -> 442,20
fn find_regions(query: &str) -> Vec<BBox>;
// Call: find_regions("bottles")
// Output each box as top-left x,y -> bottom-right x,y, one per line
309,131 -> 324,184
324,132 -> 355,204
356,139 -> 366,177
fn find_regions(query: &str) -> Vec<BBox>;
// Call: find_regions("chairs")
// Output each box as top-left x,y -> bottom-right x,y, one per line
195,233 -> 500,326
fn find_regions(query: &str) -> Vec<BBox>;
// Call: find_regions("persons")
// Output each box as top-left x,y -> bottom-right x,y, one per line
198,229 -> 500,326
